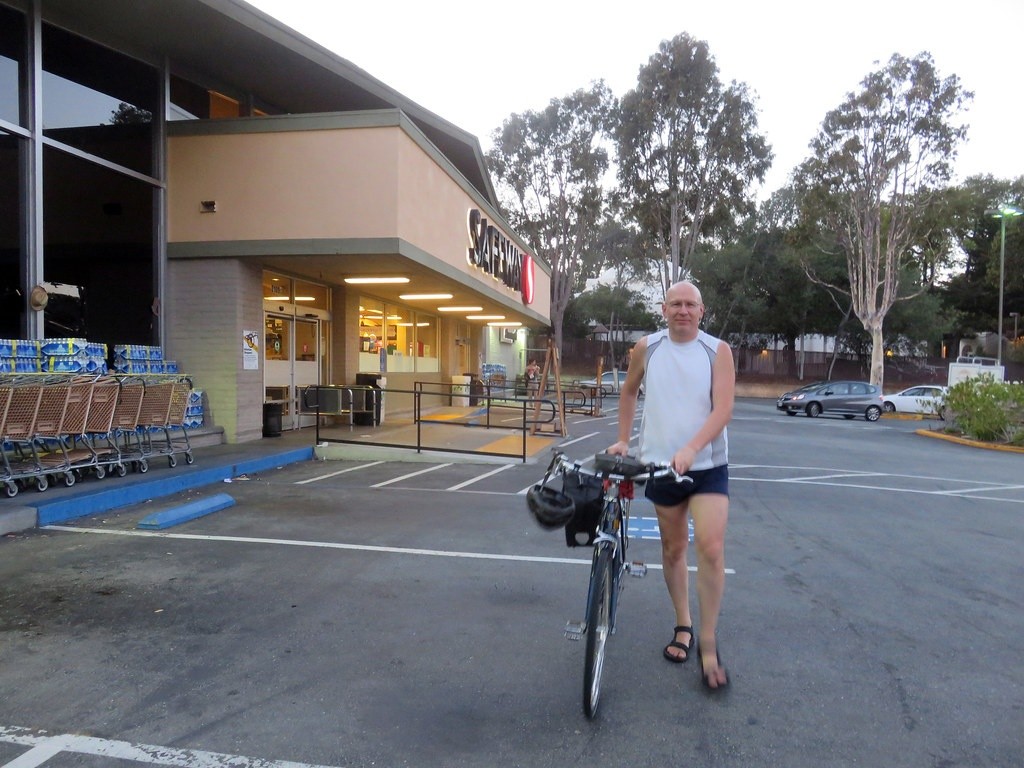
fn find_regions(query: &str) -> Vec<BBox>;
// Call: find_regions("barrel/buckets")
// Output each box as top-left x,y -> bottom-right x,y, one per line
264,403 -> 282,437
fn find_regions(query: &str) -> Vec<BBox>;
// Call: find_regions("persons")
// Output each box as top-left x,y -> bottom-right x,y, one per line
524,358 -> 541,410
604,281 -> 739,694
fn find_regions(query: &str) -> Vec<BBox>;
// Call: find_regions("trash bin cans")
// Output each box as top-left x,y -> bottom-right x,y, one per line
354,372 -> 382,426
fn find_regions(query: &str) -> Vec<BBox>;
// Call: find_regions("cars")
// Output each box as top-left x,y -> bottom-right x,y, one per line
880,383 -> 954,417
776,379 -> 886,421
580,371 -> 644,399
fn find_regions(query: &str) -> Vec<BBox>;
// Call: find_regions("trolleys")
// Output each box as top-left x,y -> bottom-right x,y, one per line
0,372 -> 198,498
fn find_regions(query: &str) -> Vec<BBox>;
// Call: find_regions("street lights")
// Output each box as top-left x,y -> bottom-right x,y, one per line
990,202 -> 1023,364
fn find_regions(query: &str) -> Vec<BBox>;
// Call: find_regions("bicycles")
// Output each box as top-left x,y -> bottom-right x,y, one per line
545,445 -> 694,719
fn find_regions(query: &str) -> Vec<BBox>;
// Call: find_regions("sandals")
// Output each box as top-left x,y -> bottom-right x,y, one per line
663,625 -> 695,664
697,637 -> 731,693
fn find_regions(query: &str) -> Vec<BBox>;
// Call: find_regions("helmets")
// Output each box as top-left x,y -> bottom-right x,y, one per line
525,484 -> 575,534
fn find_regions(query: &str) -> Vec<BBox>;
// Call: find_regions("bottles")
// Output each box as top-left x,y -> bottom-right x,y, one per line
113,344 -> 204,437
0,337 -> 109,376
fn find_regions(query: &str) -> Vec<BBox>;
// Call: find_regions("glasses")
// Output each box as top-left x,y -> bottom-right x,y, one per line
666,299 -> 701,309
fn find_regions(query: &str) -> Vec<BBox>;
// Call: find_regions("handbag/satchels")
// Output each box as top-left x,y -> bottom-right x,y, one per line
561,468 -> 604,533
